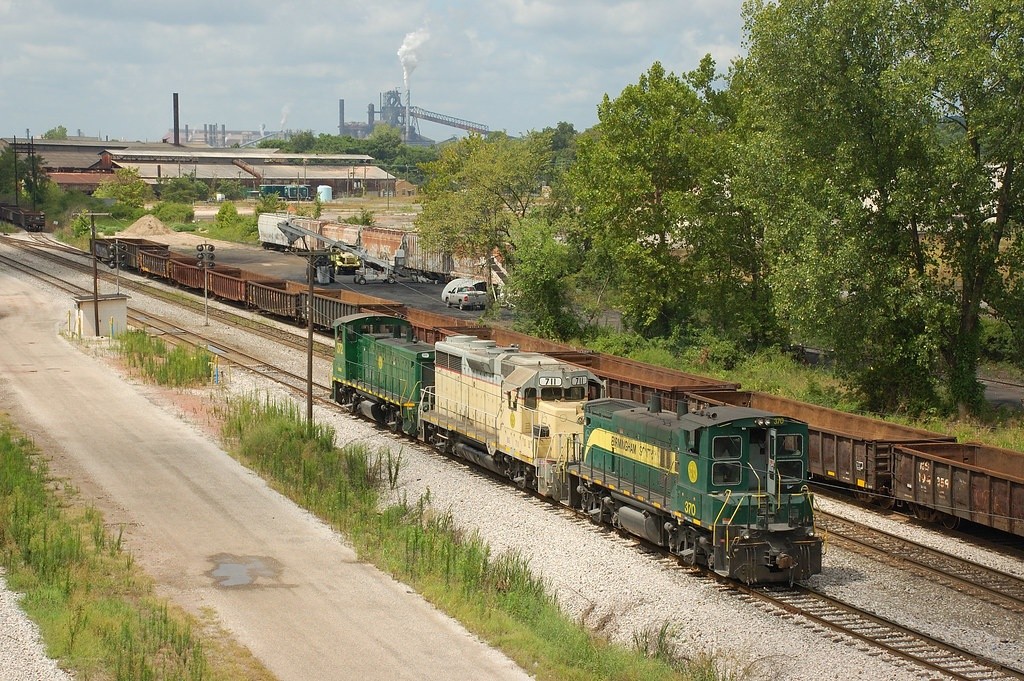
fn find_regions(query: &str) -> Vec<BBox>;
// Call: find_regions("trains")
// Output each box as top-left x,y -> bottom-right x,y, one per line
0,204 -> 1024,537
329,312 -> 825,586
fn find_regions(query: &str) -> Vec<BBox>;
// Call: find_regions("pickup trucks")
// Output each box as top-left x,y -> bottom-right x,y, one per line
445,286 -> 486,310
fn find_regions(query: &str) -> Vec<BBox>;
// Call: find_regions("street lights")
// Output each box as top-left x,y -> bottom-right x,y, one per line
109,239 -> 128,295
197,240 -> 215,326
